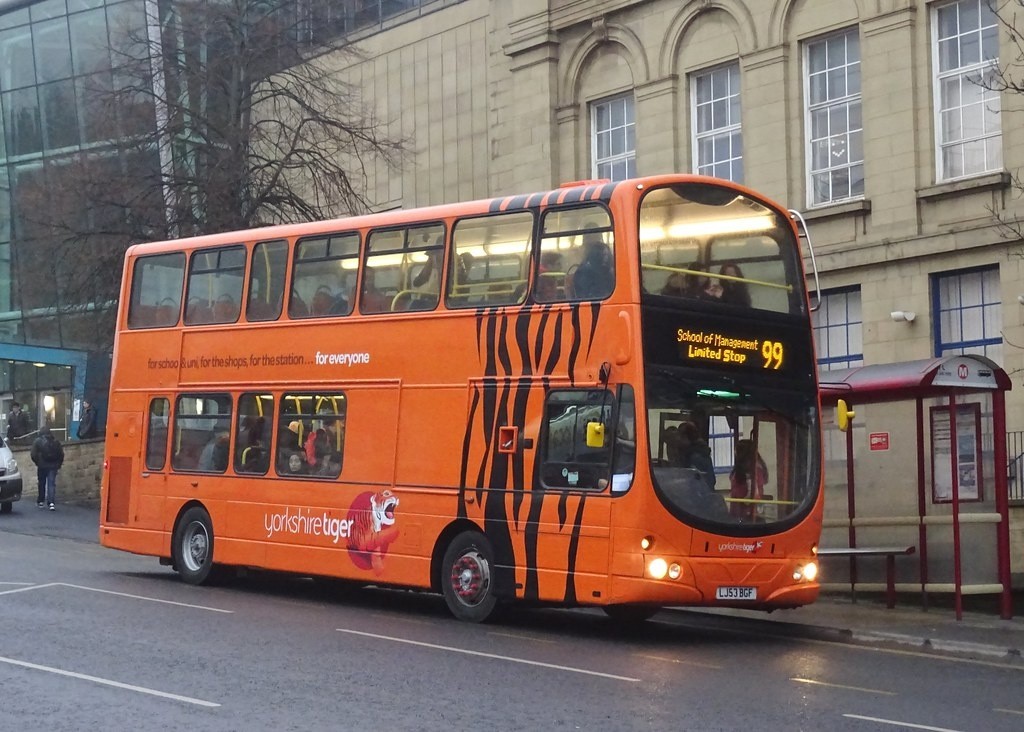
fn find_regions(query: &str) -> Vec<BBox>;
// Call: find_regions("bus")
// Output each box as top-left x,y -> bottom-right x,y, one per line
97,173 -> 828,625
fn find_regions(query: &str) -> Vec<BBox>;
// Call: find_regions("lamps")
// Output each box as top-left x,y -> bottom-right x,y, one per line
1017,295 -> 1024,306
891,311 -> 915,322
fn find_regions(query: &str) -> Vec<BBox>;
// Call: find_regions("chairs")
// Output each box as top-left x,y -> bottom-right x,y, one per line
563,264 -> 580,299
241,446 -> 270,473
155,283 -> 409,325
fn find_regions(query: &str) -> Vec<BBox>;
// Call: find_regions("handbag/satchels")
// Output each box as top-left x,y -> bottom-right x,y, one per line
416,254 -> 438,295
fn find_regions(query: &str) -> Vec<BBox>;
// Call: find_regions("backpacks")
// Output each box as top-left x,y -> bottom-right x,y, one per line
41,436 -> 61,461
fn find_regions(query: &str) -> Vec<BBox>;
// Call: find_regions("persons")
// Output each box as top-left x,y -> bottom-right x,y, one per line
663,264 -> 752,305
196,416 -> 341,476
539,252 -> 562,301
664,423 -> 716,488
728,439 -> 767,521
30,426 -> 64,511
76,400 -> 96,440
567,424 -> 630,489
6,402 -> 28,444
409,236 -> 514,310
349,266 -> 383,314
568,223 -> 614,298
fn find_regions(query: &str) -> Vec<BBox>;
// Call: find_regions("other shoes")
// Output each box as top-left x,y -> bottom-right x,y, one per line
48,502 -> 55,509
34,502 -> 44,508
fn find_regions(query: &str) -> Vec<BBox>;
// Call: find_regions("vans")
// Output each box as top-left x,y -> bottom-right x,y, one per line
0,434 -> 23,515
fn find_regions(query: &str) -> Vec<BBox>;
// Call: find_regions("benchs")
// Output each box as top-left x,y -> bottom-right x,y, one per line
817,546 -> 916,609
148,427 -> 212,471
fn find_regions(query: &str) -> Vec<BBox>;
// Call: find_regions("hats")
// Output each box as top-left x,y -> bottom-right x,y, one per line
39,426 -> 50,434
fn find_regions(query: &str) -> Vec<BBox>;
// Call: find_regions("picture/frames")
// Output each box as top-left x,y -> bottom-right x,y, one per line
929,402 -> 985,505
